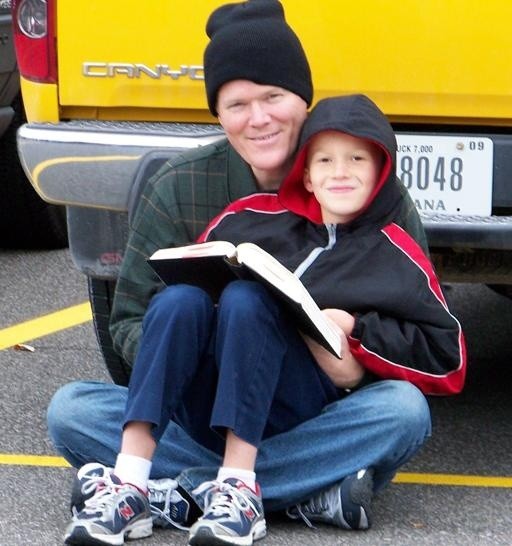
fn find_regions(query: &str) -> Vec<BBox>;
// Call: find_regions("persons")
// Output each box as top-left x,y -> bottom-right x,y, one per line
45,0 -> 433,532
61,93 -> 469,546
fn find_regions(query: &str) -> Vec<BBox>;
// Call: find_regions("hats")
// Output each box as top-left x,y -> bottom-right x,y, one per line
203,0 -> 313,118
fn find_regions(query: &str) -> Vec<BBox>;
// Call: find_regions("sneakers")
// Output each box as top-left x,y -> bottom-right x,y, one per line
285,467 -> 375,531
62,461 -> 268,545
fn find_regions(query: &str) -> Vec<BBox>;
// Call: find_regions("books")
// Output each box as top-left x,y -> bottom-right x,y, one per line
145,239 -> 343,362
